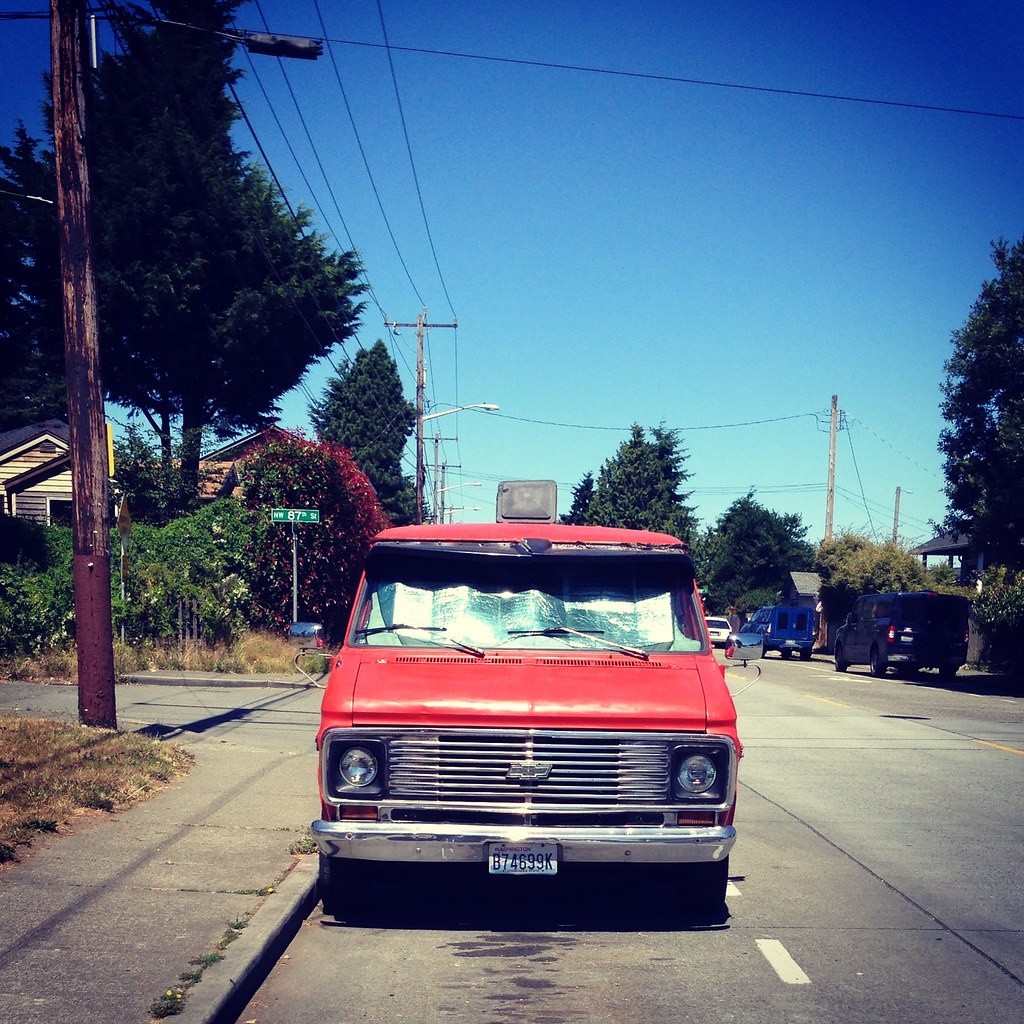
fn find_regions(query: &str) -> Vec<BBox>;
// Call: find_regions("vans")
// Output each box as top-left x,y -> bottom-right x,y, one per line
739,605 -> 816,662
288,476 -> 764,915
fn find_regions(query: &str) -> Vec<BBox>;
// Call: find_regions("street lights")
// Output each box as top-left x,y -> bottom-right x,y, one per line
432,483 -> 483,525
415,403 -> 500,526
440,506 -> 482,524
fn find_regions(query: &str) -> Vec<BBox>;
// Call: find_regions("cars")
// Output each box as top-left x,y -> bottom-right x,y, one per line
703,616 -> 733,649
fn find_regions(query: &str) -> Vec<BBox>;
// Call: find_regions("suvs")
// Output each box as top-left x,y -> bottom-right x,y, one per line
833,589 -> 971,678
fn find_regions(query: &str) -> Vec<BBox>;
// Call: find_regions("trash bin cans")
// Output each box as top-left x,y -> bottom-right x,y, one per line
289,622 -> 323,651
